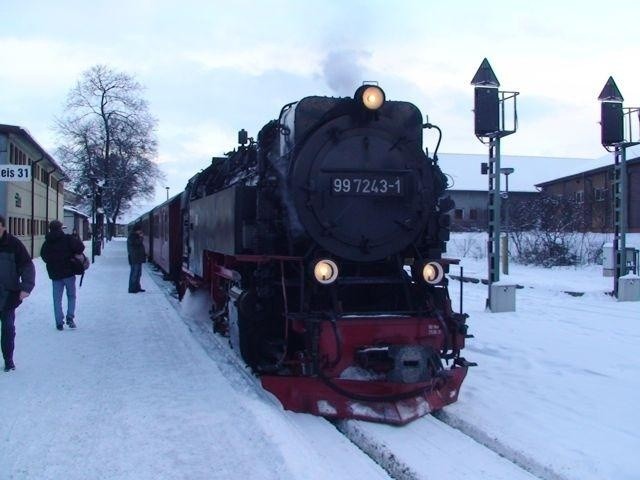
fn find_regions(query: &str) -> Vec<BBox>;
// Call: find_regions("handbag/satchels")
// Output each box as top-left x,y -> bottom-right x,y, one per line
65,252 -> 90,287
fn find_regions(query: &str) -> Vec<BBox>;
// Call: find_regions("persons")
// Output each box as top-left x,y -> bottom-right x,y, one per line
0,214 -> 36,373
38,219 -> 86,329
71,227 -> 80,240
127,223 -> 146,294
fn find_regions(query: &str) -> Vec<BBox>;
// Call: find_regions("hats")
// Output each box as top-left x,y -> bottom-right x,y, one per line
50,219 -> 67,229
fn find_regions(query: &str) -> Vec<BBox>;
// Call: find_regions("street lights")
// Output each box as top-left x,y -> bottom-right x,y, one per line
86,174 -> 99,264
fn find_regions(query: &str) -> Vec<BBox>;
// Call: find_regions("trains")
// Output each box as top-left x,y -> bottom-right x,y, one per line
123,78 -> 475,426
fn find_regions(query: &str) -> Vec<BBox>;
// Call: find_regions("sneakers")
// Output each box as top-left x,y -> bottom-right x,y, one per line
57,325 -> 63,330
4,360 -> 16,372
129,289 -> 146,293
64,318 -> 78,328
